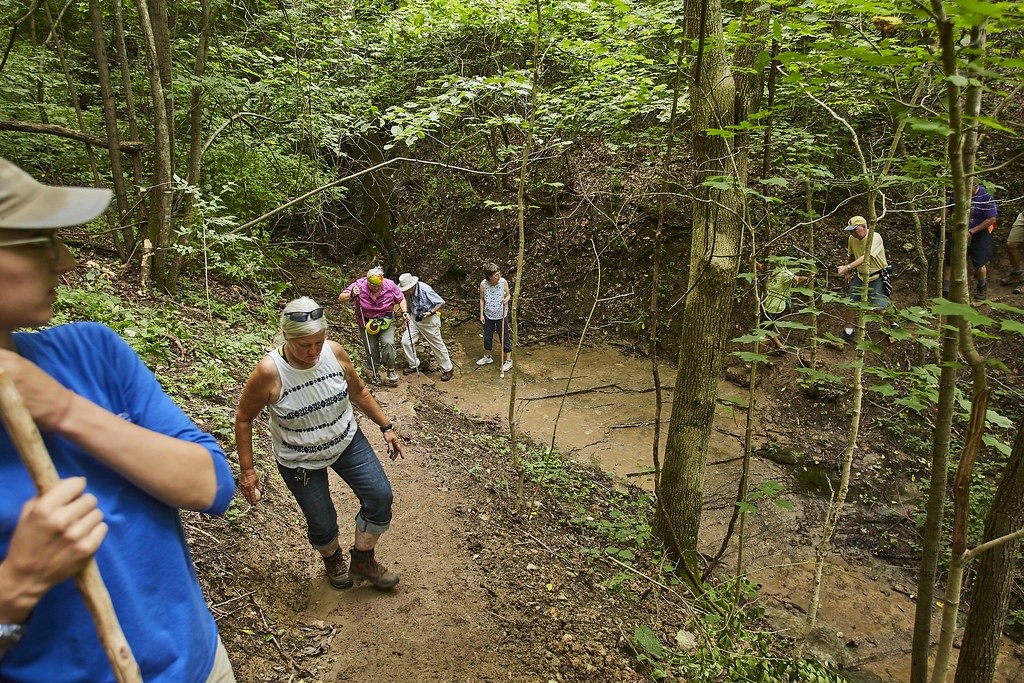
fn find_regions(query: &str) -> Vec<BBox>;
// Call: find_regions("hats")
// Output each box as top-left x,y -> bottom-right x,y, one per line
0,153 -> 113,229
397,272 -> 418,292
845,216 -> 867,230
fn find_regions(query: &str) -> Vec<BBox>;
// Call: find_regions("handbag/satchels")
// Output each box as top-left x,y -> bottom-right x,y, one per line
880,264 -> 893,296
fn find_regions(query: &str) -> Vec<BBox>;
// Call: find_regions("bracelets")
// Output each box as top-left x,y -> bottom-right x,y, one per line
846,264 -> 852,271
429,310 -> 433,314
968,230 -> 973,235
401,311 -> 409,314
380,424 -> 395,432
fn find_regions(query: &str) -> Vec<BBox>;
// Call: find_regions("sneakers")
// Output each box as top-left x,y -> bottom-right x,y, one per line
325,548 -> 354,588
500,359 -> 513,371
476,354 -> 496,366
348,557 -> 399,587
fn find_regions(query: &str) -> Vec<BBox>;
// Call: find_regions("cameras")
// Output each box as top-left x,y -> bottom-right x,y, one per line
415,306 -> 429,322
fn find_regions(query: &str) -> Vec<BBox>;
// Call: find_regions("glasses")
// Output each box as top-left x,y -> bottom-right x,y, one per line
0,232 -> 59,261
285,307 -> 325,322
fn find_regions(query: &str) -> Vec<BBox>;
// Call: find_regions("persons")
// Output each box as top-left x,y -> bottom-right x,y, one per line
235,295 -> 405,590
744,250 -> 816,369
0,156 -> 236,683
397,273 -> 454,381
1001,209 -> 1024,295
338,266 -> 410,383
836,216 -> 901,340
476,262 -> 514,372
931,176 -> 998,300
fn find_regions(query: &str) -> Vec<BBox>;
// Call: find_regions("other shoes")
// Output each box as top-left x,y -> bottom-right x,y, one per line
441,368 -> 455,381
372,372 -> 381,384
388,368 -> 396,384
974,280 -> 986,300
767,348 -> 788,356
1014,281 -> 1024,294
840,329 -> 853,341
1001,276 -> 1020,284
405,363 -> 422,373
